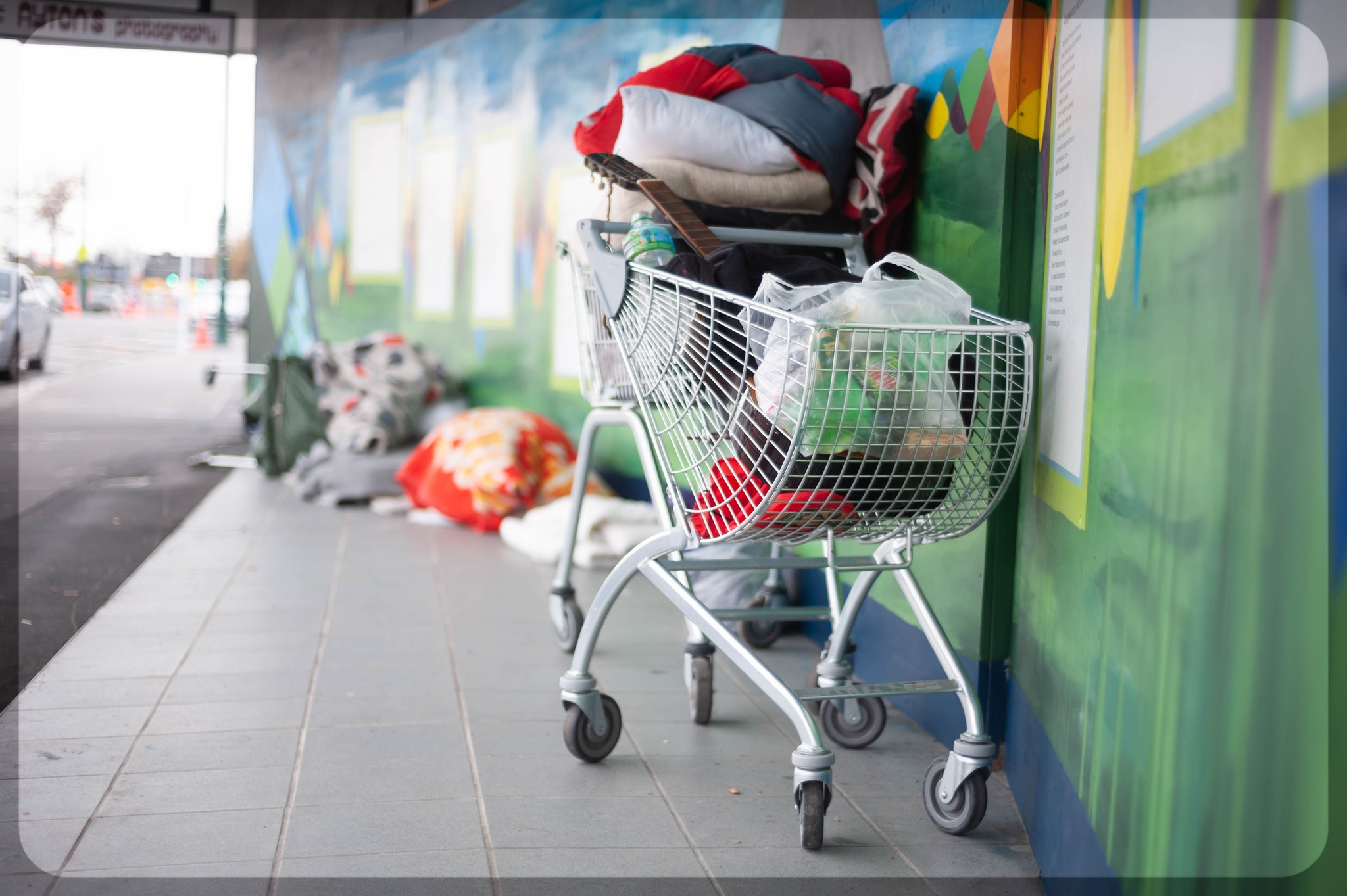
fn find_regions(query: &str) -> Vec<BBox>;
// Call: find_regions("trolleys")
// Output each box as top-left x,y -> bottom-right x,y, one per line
543,217 -> 1032,853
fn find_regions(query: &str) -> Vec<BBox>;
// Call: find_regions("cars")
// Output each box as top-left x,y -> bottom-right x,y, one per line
33,274 -> 64,314
0,260 -> 49,377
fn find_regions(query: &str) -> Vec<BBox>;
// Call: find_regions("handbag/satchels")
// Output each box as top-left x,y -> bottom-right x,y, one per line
734,252 -> 973,460
